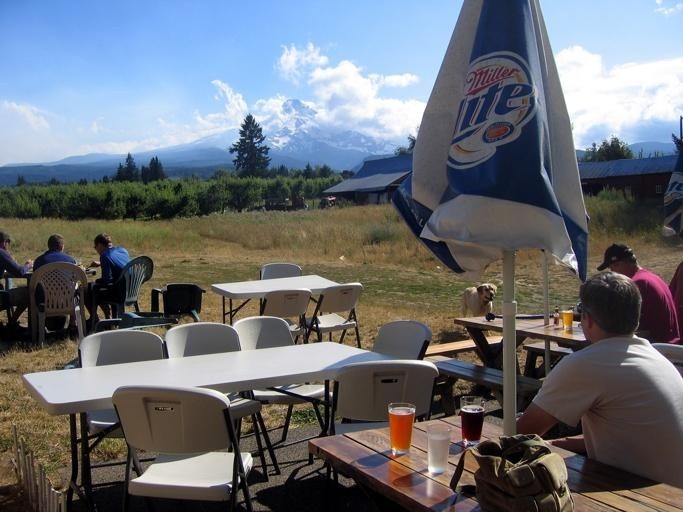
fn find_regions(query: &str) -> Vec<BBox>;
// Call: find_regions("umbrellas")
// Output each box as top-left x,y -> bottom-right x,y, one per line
662,140 -> 682,238
389,1 -> 593,434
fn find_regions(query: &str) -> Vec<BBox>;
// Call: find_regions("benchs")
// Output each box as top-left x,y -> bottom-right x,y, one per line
424,335 -> 502,363
432,353 -> 543,414
522,342 -> 573,377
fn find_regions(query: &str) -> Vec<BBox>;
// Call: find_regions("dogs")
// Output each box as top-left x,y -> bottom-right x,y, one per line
461,283 -> 497,337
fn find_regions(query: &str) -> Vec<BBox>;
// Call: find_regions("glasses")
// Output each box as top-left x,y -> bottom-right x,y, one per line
577,300 -> 594,320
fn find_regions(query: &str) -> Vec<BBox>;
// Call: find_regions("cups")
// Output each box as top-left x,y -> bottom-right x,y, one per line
460,396 -> 486,445
426,423 -> 453,474
562,310 -> 573,330
388,402 -> 416,455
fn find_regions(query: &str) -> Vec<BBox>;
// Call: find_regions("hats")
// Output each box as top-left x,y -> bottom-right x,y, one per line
597,243 -> 633,271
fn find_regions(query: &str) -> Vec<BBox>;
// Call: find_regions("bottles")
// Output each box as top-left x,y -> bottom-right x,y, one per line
554,309 -> 560,329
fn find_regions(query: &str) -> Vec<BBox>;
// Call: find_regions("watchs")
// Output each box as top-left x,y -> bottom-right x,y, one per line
516,411 -> 524,418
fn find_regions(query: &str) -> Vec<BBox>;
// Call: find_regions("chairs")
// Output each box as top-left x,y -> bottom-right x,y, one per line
651,343 -> 683,378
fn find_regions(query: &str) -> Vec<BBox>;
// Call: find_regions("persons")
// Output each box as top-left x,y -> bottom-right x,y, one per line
78,233 -> 132,323
596,242 -> 682,344
33,233 -> 78,341
515,271 -> 683,490
0,230 -> 34,333
669,261 -> 683,345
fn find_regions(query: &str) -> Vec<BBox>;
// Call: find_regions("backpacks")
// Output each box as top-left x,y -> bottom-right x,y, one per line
449,433 -> 574,512
